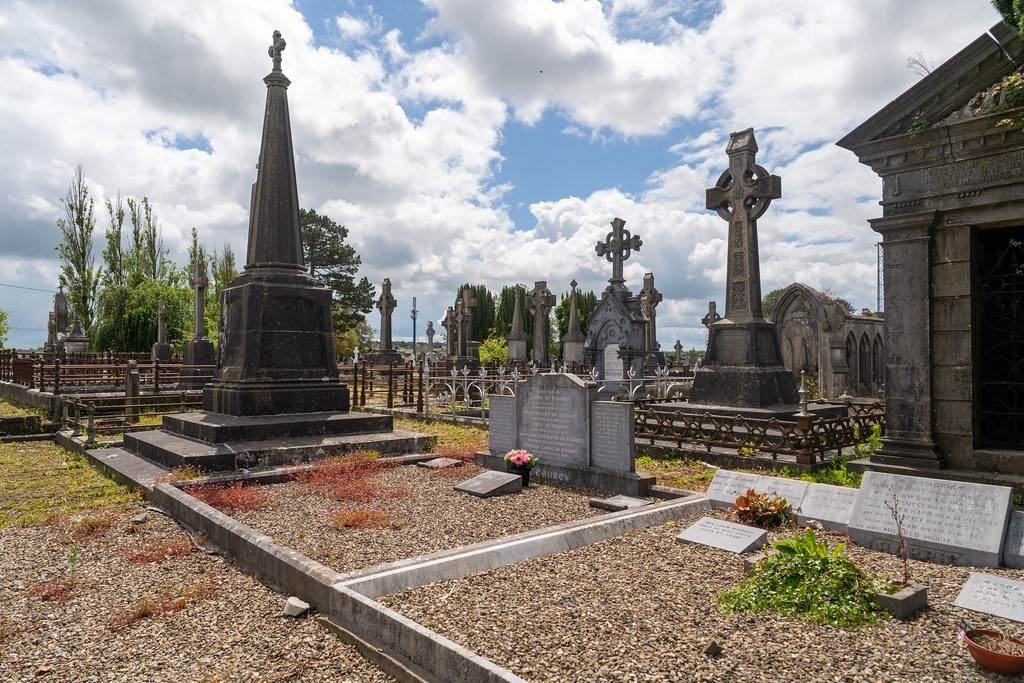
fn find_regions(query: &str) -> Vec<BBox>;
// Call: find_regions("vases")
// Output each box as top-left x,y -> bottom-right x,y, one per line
963,629 -> 1024,676
507,468 -> 531,486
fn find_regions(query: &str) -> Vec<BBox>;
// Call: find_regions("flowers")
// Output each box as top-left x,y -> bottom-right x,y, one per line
504,448 -> 539,469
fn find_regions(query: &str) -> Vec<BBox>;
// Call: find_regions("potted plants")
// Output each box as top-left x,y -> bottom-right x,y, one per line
737,488 -> 791,526
